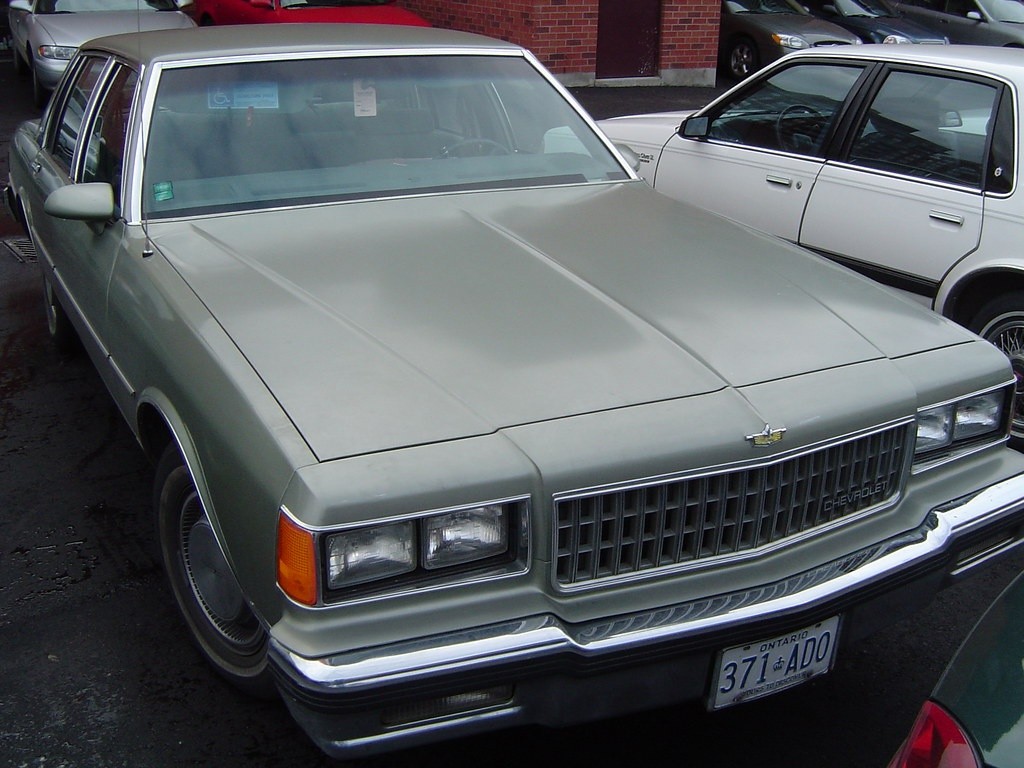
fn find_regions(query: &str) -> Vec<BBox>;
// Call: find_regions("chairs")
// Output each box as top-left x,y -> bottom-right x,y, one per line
155,97 -> 430,179
851,97 -> 958,173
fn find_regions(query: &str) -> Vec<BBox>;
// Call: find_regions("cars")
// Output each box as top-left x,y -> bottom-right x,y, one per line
718,0 -> 865,85
883,0 -> 1024,49
800,0 -> 954,50
537,39 -> 1024,456
183,0 -> 434,29
889,570 -> 1024,768
5,0 -> 204,111
4,19 -> 1024,760
